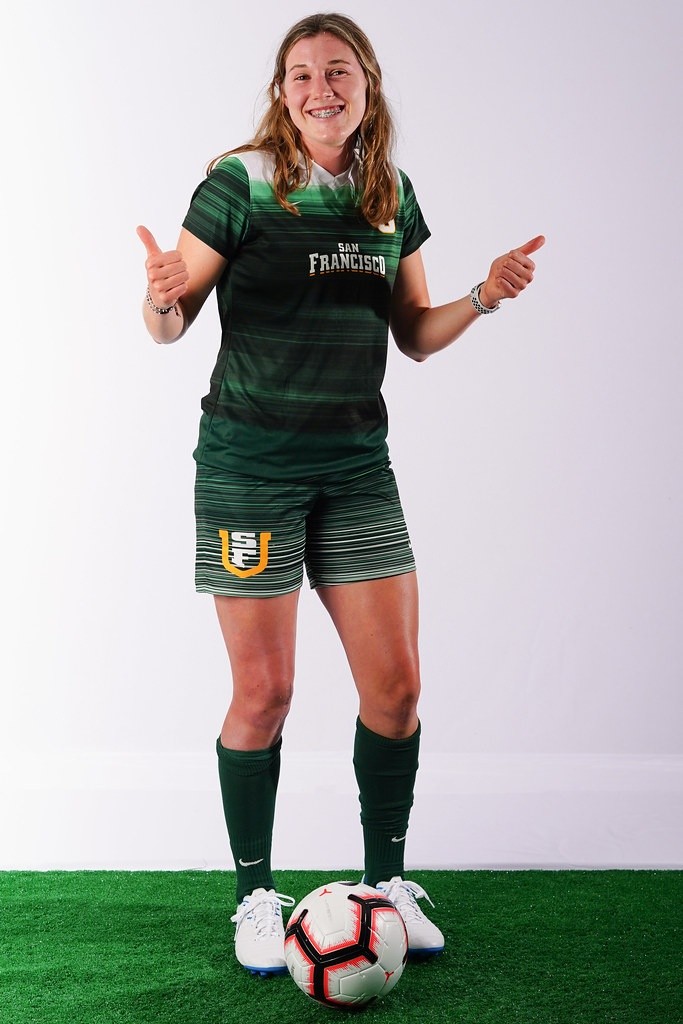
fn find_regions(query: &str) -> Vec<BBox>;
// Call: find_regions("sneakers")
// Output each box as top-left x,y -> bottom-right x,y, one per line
360,874 -> 445,952
230,888 -> 296,977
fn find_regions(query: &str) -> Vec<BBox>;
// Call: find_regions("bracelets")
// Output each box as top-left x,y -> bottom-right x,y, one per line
145,287 -> 179,316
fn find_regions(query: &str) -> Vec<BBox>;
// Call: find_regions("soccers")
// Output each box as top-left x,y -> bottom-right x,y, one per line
284,881 -> 408,1009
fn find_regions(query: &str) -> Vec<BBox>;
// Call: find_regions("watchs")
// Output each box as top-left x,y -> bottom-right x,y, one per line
471,280 -> 502,315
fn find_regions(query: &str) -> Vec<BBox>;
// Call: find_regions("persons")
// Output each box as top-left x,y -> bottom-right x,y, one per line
133,14 -> 544,979
